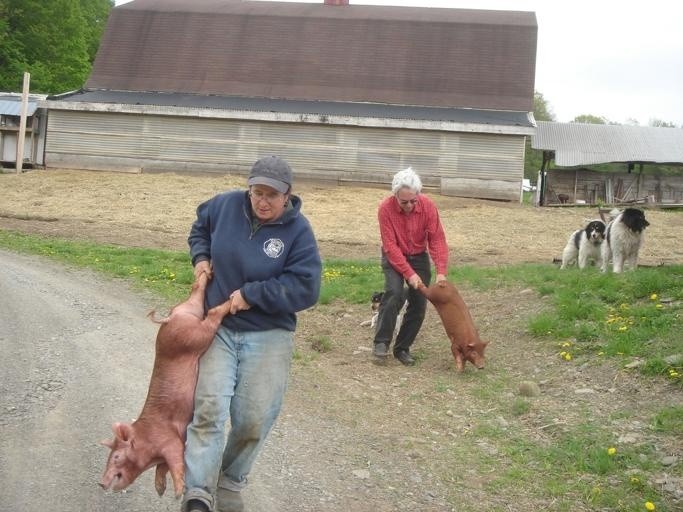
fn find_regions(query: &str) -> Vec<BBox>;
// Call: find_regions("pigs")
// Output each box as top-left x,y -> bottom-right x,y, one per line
98,269 -> 233,500
417,277 -> 490,373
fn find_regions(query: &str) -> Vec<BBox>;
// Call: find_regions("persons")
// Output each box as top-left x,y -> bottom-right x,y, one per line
372,166 -> 449,366
178,156 -> 323,512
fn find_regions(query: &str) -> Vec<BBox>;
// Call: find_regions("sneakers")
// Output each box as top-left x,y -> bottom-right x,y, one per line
394,350 -> 415,365
217,486 -> 243,511
373,343 -> 388,356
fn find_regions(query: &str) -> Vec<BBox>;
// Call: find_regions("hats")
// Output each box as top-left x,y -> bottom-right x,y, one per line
248,156 -> 292,193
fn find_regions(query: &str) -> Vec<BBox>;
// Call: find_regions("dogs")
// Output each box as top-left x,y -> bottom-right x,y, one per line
599,207 -> 650,274
559,219 -> 606,271
359,291 -> 410,335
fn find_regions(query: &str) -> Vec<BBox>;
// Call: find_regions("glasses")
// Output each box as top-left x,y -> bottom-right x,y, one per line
400,200 -> 417,204
249,187 -> 281,199
